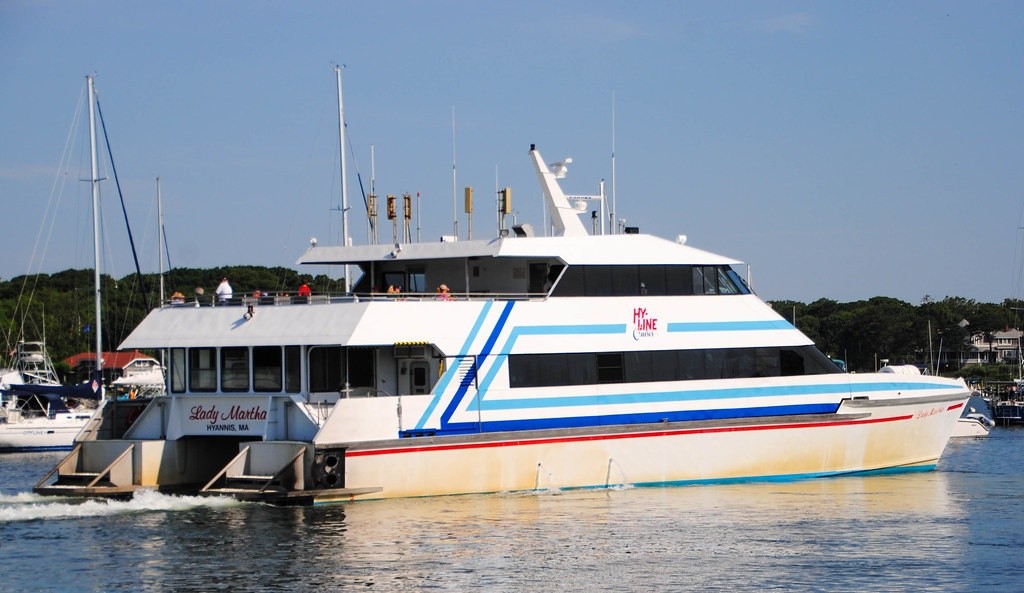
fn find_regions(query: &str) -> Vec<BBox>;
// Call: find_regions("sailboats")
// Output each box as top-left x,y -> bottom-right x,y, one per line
0,69 -> 180,454
979,327 -> 1024,423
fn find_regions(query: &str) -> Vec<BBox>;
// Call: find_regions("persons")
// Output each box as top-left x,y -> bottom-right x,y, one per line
187,287 -> 210,306
387,281 -> 401,299
215,277 -> 233,306
435,285 -> 450,301
297,279 -> 311,304
253,290 -> 289,305
170,292 -> 185,304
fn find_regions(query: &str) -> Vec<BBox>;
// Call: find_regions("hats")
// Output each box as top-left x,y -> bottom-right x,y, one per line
440,284 -> 449,290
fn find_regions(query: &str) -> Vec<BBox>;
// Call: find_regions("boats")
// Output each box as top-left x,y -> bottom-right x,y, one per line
31,60 -> 974,508
949,418 -> 991,437
